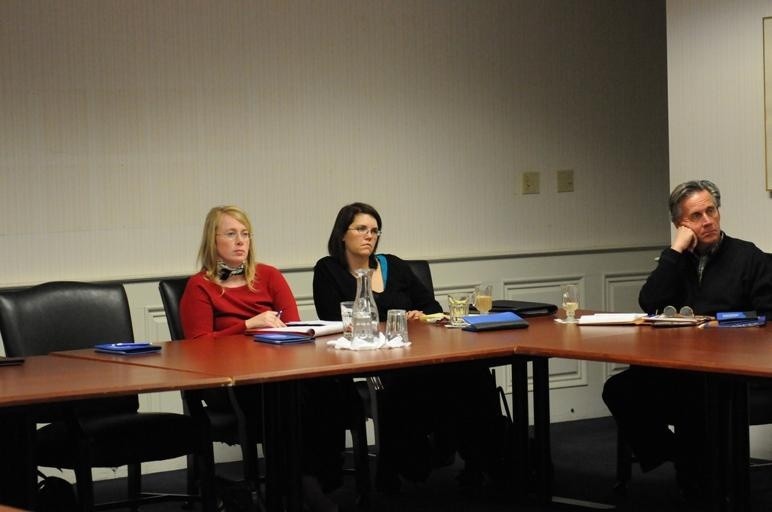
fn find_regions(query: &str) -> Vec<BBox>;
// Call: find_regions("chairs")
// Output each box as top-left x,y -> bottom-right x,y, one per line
156,280 -> 369,512
354,260 -> 496,471
0,281 -> 187,512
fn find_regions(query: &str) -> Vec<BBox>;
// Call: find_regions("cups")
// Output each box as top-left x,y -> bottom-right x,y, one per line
449,293 -> 470,325
341,302 -> 358,339
384,309 -> 409,344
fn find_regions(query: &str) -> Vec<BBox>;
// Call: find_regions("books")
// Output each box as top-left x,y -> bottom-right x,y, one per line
95,341 -> 163,356
244,318 -> 350,339
716,310 -> 759,325
253,333 -> 311,345
461,311 -> 527,332
640,312 -> 716,326
470,299 -> 557,318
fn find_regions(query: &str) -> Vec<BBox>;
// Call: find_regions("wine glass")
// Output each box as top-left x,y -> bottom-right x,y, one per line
350,268 -> 380,343
471,283 -> 493,315
559,283 -> 580,323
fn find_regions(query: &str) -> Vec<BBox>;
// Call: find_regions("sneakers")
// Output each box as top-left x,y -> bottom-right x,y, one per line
301,480 -> 338,511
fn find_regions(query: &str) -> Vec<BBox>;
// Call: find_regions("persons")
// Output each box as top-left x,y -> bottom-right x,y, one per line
603,180 -> 772,487
314,203 -> 502,492
179,205 -> 347,512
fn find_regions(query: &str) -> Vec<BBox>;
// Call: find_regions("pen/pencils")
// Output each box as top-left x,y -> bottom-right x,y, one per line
276,310 -> 283,316
116,344 -> 151,347
287,324 -> 326,326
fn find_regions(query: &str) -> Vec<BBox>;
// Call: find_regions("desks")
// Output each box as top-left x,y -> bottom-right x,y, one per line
516,321 -> 772,512
59,309 -> 630,512
0,354 -> 230,511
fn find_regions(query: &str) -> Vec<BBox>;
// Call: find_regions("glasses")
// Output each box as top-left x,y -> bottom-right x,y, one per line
216,231 -> 250,240
656,305 -> 694,318
347,226 -> 383,237
